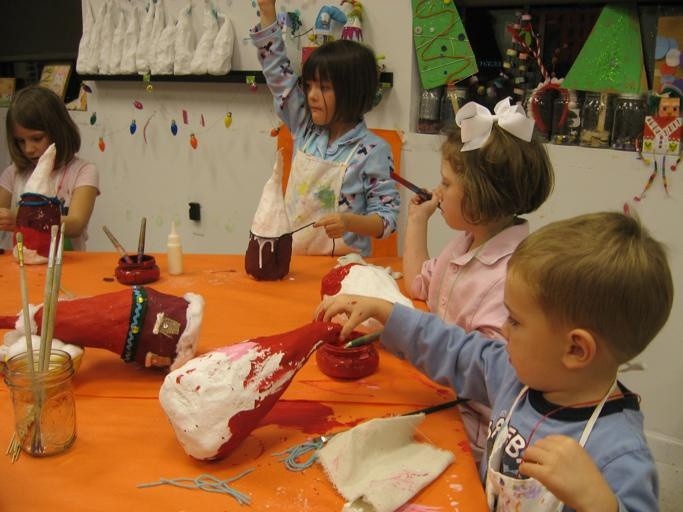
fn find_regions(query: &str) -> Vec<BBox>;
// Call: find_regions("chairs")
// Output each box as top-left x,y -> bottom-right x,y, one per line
276,123 -> 402,262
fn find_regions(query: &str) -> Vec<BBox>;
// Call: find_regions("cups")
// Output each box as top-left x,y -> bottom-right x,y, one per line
4,348 -> 78,458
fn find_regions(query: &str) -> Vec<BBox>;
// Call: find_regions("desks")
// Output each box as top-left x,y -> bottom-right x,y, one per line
1,247 -> 488,512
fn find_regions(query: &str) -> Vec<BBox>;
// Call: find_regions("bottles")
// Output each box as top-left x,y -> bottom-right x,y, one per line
168,221 -> 183,278
417,82 -> 466,136
526,89 -> 647,153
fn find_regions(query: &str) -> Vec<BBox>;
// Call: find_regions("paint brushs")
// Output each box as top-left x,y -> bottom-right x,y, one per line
343,332 -> 381,349
137,217 -> 146,264
402,399 -> 472,416
102,226 -> 133,265
16,222 -> 66,456
390,172 -> 441,209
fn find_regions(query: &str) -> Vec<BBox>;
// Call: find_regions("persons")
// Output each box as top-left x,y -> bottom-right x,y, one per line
249,0 -> 401,258
0,85 -> 100,251
